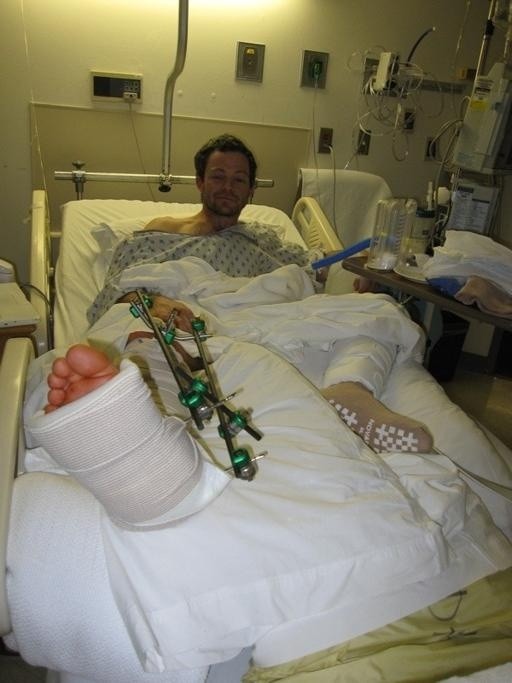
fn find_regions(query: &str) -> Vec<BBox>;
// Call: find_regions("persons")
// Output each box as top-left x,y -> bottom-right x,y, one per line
25,134 -> 433,531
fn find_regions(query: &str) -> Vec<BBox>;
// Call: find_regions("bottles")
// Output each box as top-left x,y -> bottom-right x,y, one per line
402,202 -> 438,263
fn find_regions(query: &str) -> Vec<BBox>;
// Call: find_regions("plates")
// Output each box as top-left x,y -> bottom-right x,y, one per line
393,264 -> 448,285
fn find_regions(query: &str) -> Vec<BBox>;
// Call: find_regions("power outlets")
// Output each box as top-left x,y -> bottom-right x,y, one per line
317,128 -> 333,155
357,129 -> 371,155
234,41 -> 265,84
299,48 -> 329,88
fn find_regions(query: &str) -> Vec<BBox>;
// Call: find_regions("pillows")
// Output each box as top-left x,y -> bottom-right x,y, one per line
89,216 -> 150,265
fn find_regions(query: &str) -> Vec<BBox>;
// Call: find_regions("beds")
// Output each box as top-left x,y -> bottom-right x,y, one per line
0,189 -> 511,682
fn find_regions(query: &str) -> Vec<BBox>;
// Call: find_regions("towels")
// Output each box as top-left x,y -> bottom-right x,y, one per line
454,275 -> 512,321
